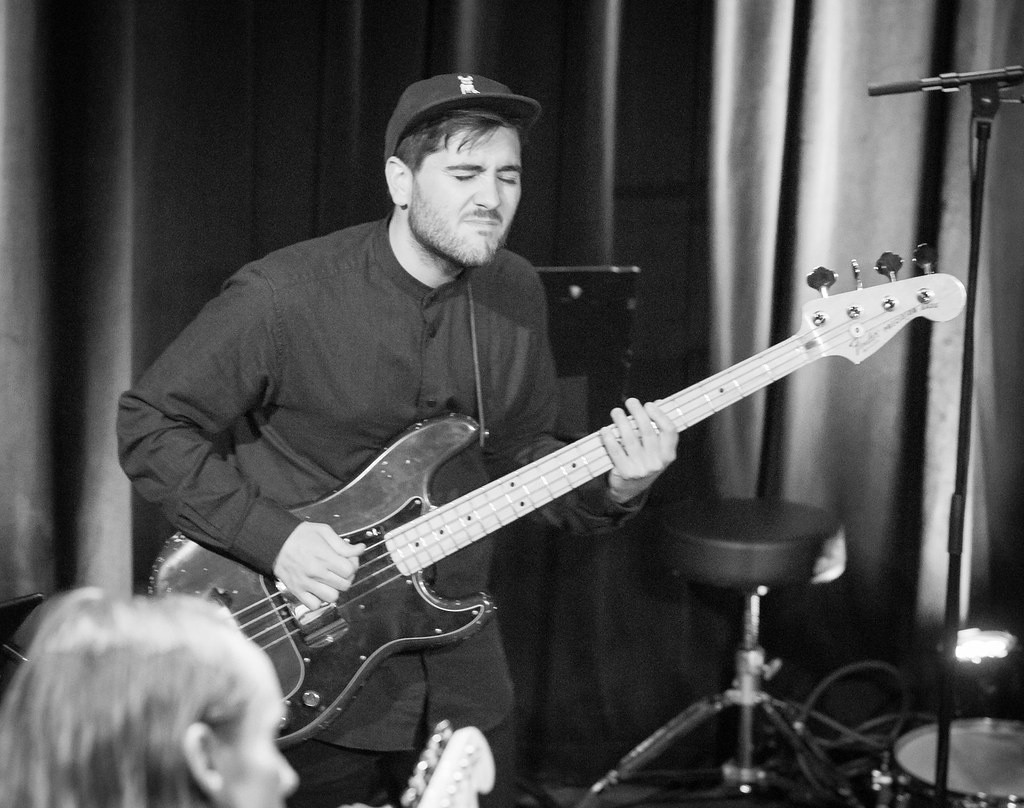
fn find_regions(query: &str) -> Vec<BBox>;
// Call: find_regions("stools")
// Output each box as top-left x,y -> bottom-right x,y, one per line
579,498 -> 888,808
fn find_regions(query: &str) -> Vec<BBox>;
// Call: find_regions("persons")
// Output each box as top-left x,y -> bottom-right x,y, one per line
116,74 -> 677,808
0,587 -> 299,807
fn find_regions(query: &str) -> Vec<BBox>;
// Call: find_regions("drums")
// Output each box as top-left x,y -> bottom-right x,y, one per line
893,716 -> 1023,807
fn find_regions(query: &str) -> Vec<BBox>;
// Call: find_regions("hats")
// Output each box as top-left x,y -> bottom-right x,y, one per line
384,74 -> 542,171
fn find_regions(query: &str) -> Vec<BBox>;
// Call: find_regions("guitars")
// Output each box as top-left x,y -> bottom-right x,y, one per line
146,240 -> 969,748
401,717 -> 496,808
